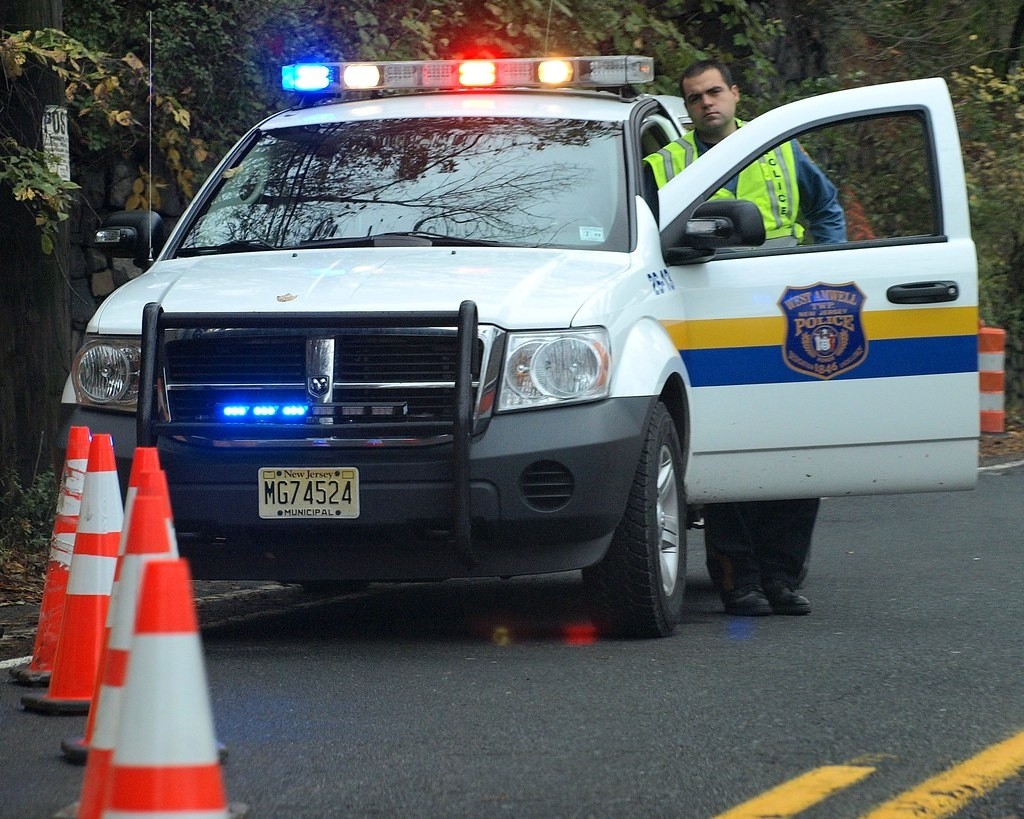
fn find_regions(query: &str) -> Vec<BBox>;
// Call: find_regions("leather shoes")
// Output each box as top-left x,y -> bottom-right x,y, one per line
724,591 -> 773,615
766,588 -> 812,613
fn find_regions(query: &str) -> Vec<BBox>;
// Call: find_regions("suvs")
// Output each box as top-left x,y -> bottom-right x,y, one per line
50,57 -> 982,641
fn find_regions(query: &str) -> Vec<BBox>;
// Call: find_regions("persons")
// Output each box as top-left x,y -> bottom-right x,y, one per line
641,59 -> 849,617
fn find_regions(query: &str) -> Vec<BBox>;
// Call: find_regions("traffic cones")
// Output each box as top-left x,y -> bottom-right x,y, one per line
9,426 -> 93,684
62,447 -> 231,764
19,434 -> 124,715
76,495 -> 172,819
99,556 -> 232,819
